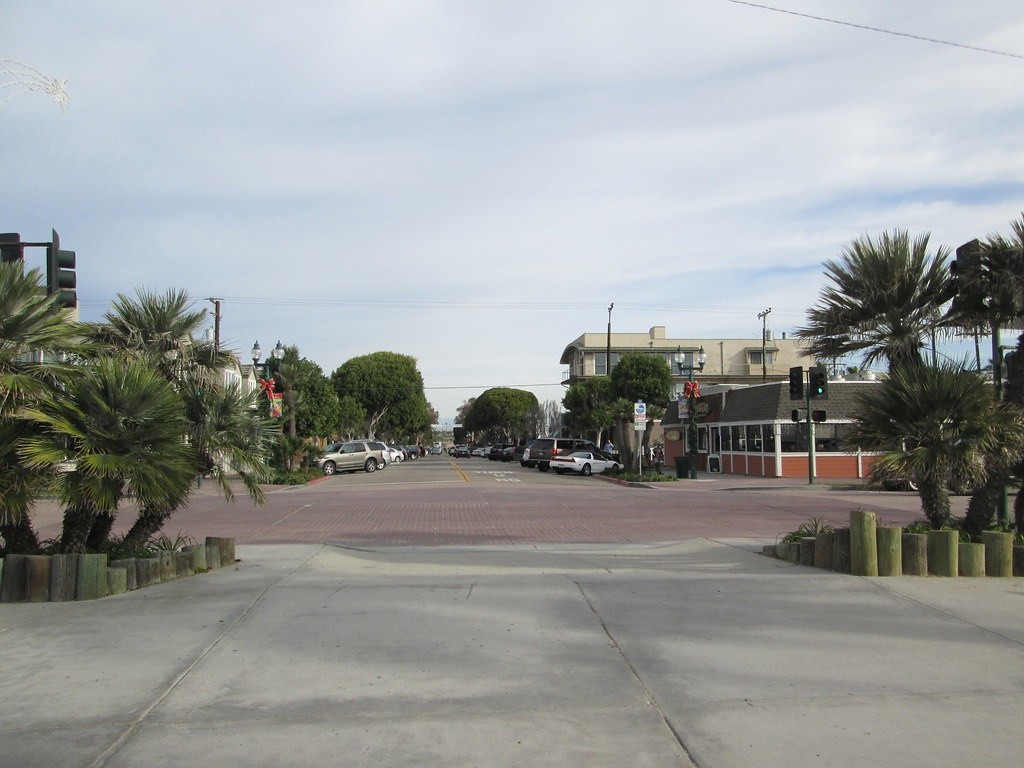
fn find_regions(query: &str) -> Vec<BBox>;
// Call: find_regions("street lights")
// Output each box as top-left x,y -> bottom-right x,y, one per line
249,339 -> 285,484
674,344 -> 707,481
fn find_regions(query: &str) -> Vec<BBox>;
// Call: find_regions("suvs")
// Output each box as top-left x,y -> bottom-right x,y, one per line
520,442 -> 541,468
528,436 -> 596,473
301,441 -> 385,476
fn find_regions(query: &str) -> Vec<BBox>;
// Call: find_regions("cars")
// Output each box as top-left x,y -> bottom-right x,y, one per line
548,448 -> 620,476
350,439 -> 517,477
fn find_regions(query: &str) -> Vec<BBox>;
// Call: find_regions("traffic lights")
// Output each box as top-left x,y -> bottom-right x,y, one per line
792,409 -> 805,423
49,228 -> 77,316
789,366 -> 804,401
813,410 -> 826,421
809,366 -> 829,400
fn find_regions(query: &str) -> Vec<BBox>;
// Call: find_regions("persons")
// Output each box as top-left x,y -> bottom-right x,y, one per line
604,440 -> 614,455
654,444 -> 664,475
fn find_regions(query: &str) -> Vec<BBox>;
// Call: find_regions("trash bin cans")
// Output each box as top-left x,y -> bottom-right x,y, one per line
673,456 -> 690,478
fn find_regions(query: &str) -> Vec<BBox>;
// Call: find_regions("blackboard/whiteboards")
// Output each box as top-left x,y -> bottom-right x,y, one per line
708,455 -> 720,473
